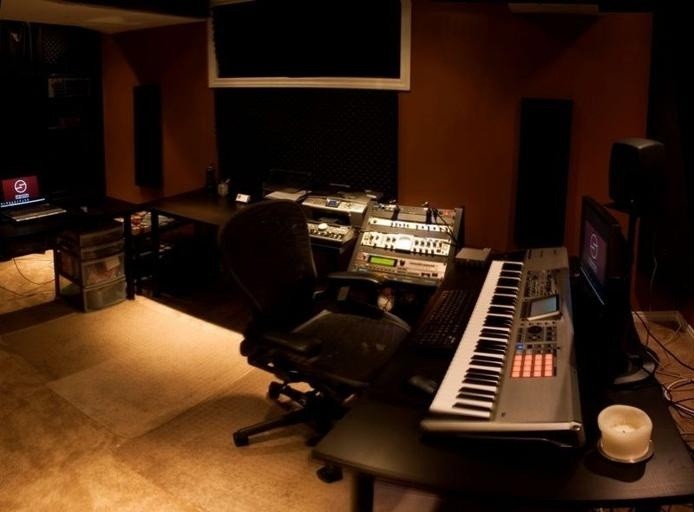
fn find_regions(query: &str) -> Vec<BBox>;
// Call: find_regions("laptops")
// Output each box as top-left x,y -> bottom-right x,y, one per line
1,173 -> 67,223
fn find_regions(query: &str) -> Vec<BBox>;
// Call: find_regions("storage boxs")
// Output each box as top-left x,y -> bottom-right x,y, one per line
58,221 -> 127,312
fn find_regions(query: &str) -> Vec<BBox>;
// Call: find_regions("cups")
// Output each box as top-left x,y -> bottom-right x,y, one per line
217,184 -> 229,196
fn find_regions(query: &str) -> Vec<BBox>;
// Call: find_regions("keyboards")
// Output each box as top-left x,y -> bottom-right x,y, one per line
410,290 -> 474,349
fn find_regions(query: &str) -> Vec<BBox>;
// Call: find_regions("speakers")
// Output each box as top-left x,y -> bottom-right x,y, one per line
132,83 -> 163,189
607,137 -> 665,208
515,98 -> 574,250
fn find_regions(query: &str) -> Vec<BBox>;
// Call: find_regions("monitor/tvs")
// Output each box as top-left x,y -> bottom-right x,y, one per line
577,196 -> 626,313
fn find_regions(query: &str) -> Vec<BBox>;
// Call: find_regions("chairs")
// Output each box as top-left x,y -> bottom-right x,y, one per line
218,199 -> 409,483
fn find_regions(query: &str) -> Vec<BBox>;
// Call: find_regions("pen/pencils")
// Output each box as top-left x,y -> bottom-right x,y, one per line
215,178 -> 231,185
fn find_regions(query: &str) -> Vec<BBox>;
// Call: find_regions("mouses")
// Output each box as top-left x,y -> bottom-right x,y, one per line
409,376 -> 436,395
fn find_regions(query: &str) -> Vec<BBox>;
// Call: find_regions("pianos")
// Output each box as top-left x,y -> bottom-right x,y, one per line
417,247 -> 589,452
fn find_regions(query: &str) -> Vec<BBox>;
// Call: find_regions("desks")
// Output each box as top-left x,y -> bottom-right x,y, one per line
149,189 -> 357,314
114,213 -> 190,295
0,196 -> 140,299
313,250 -> 694,512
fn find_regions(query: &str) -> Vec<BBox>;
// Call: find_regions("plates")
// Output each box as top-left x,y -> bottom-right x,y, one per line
598,437 -> 655,465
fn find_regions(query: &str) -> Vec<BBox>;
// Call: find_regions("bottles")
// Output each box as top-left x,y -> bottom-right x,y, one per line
207,163 -> 215,189
